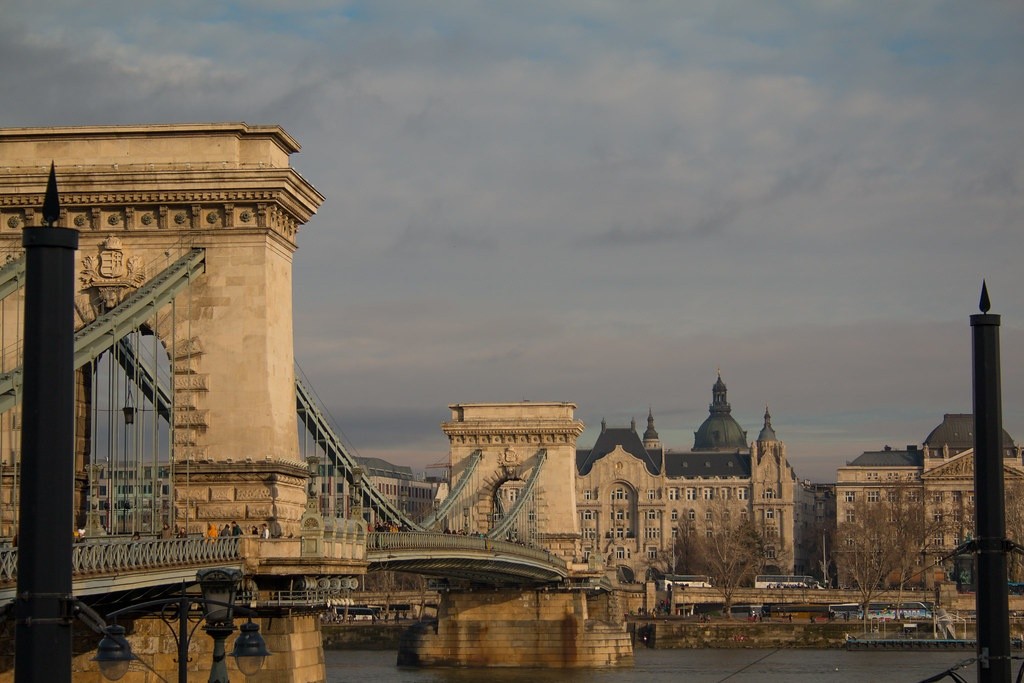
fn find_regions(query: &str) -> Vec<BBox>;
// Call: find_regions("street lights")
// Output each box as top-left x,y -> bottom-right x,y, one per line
90,575 -> 274,683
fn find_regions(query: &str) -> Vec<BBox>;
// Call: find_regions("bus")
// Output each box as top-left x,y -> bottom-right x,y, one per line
754,574 -> 820,588
721,606 -> 761,622
662,574 -> 712,588
861,601 -> 932,620
828,603 -> 860,619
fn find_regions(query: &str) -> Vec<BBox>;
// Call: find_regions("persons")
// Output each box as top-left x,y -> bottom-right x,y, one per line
14,517 -> 280,547
367,522 -> 613,567
319,610 -> 407,624
639,583 -> 899,624
